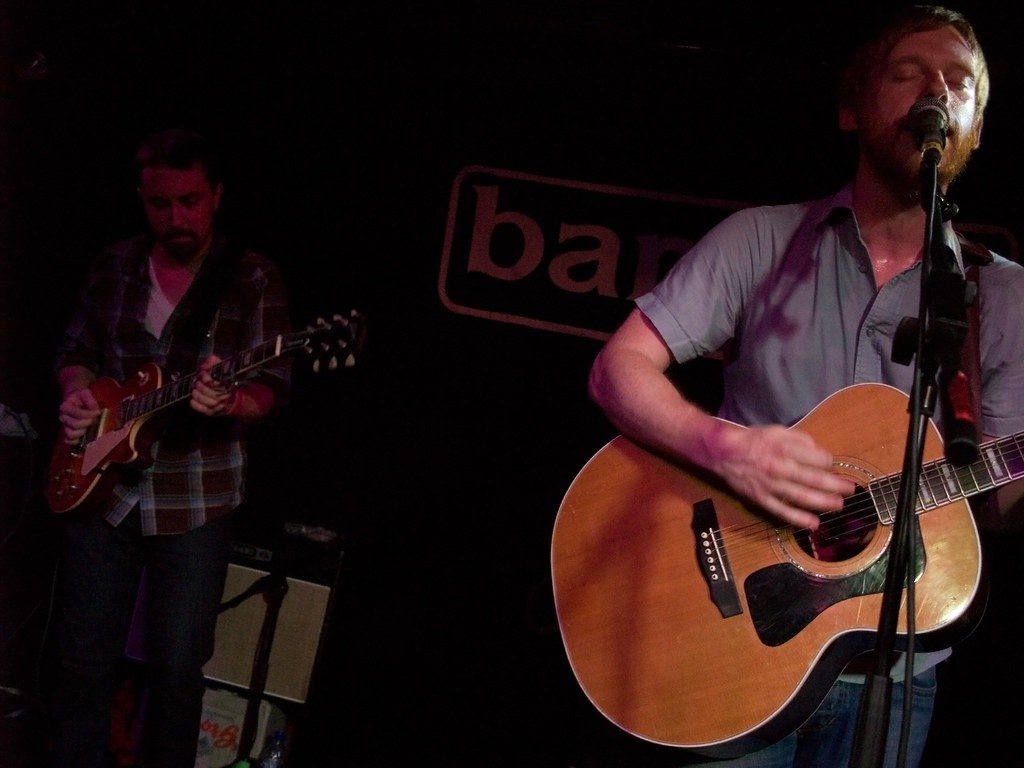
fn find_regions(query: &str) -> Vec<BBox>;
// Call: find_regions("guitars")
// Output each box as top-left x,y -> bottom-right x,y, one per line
44,307 -> 366,527
546,379 -> 1024,762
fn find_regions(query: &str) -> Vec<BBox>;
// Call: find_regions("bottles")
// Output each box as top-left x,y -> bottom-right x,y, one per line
261,731 -> 287,768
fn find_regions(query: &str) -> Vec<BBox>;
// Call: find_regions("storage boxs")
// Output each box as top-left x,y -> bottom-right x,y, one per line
112,545 -> 345,768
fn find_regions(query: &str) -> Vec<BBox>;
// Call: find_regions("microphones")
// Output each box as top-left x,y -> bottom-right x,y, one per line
907,97 -> 949,163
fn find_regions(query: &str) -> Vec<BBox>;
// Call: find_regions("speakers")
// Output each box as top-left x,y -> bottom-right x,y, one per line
123,541 -> 334,703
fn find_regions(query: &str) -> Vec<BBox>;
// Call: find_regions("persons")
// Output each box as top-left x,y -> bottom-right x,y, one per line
45,127 -> 291,767
587,6 -> 1024,768
